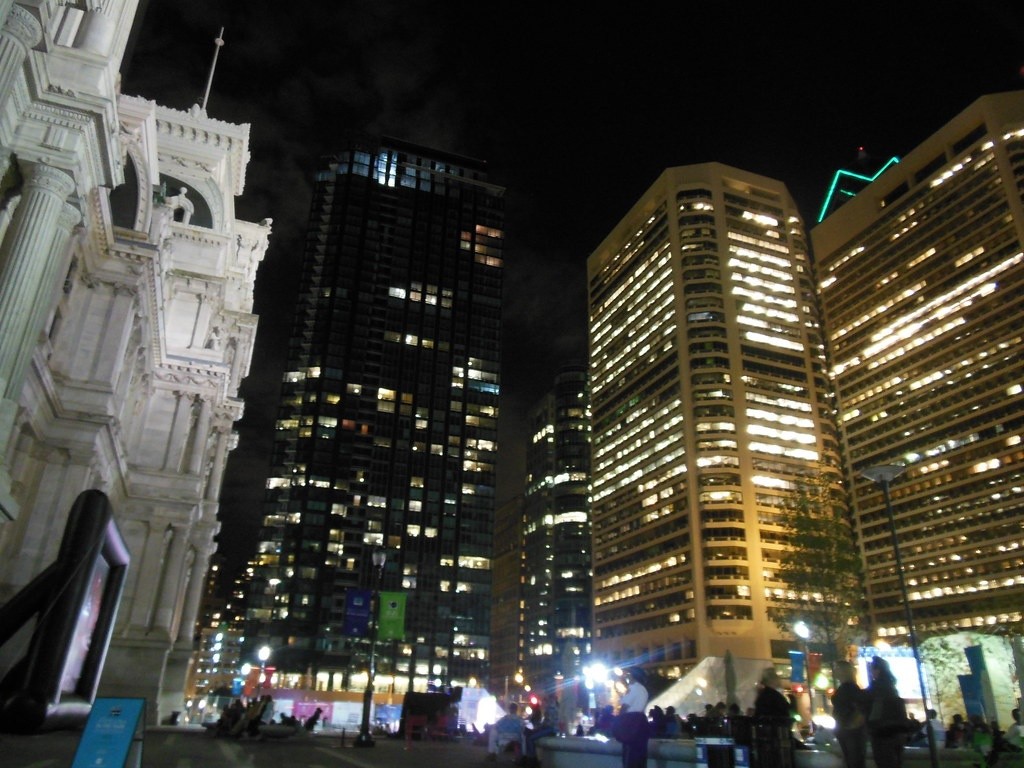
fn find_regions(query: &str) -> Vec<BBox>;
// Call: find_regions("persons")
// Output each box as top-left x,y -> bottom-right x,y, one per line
213,694 -> 302,740
486,657 -> 1024,768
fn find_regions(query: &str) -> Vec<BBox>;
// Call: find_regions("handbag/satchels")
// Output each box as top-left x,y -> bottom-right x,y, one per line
867,697 -> 910,730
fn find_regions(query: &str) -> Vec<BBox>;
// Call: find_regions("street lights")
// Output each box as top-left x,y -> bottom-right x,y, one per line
795,619 -> 814,719
353,542 -> 390,750
859,459 -> 939,768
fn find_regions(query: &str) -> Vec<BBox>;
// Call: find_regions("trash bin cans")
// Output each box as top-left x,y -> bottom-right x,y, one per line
696,715 -> 797,768
